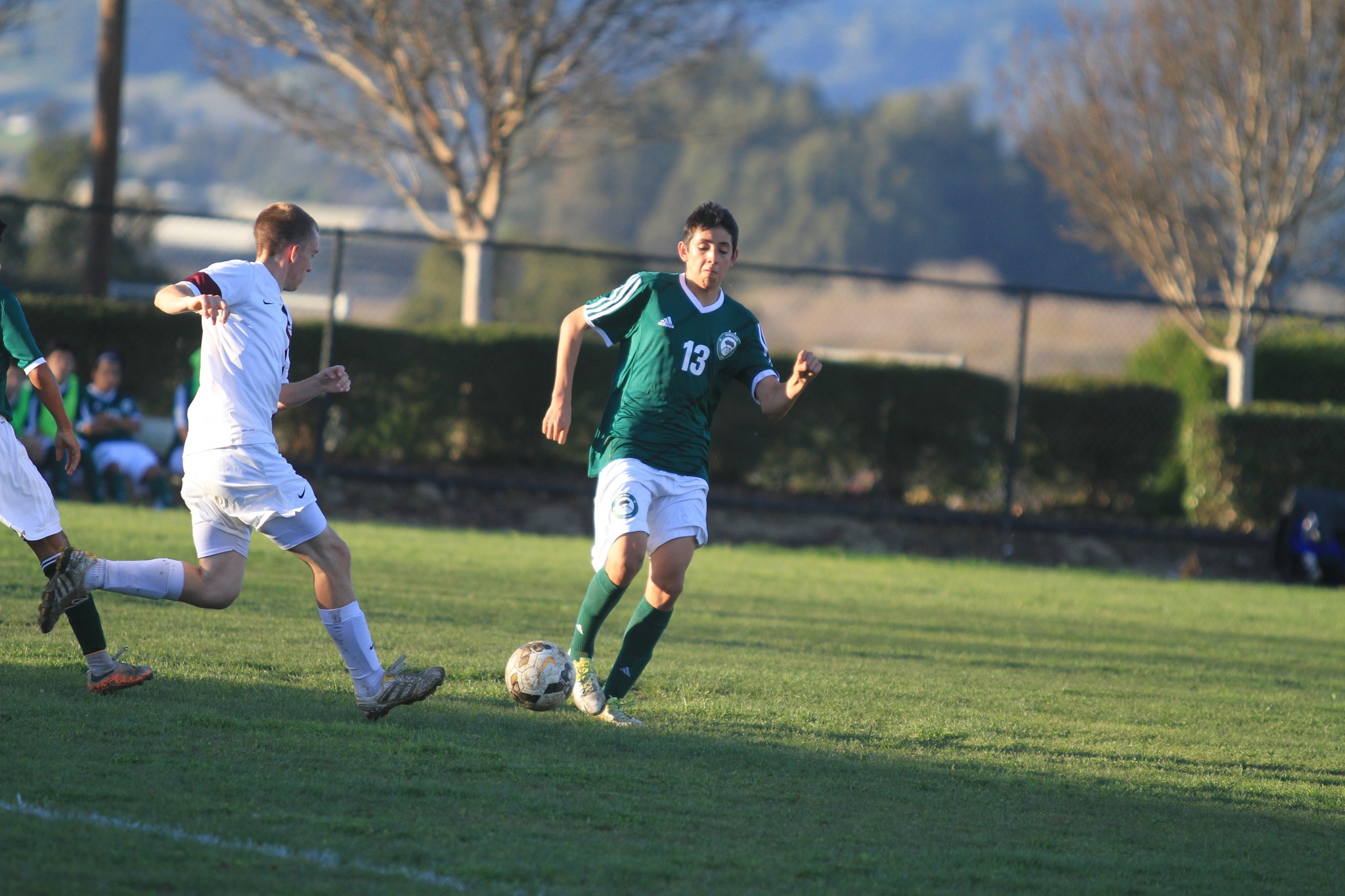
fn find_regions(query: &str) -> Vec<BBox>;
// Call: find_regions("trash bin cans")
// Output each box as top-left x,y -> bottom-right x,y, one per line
1274,488 -> 1345,586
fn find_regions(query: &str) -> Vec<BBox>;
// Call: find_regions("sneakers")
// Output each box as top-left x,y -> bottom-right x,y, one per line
567,647 -> 605,716
354,654 -> 446,721
39,548 -> 97,634
592,693 -> 643,728
87,646 -> 154,695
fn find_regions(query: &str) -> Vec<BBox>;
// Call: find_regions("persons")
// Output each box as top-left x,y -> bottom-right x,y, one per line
0,282 -> 154,695
166,344 -> 201,504
74,350 -> 163,504
540,199 -> 823,727
5,347 -> 90,500
39,202 -> 446,721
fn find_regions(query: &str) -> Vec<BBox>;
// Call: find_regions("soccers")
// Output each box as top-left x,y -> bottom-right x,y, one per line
504,640 -> 576,712
